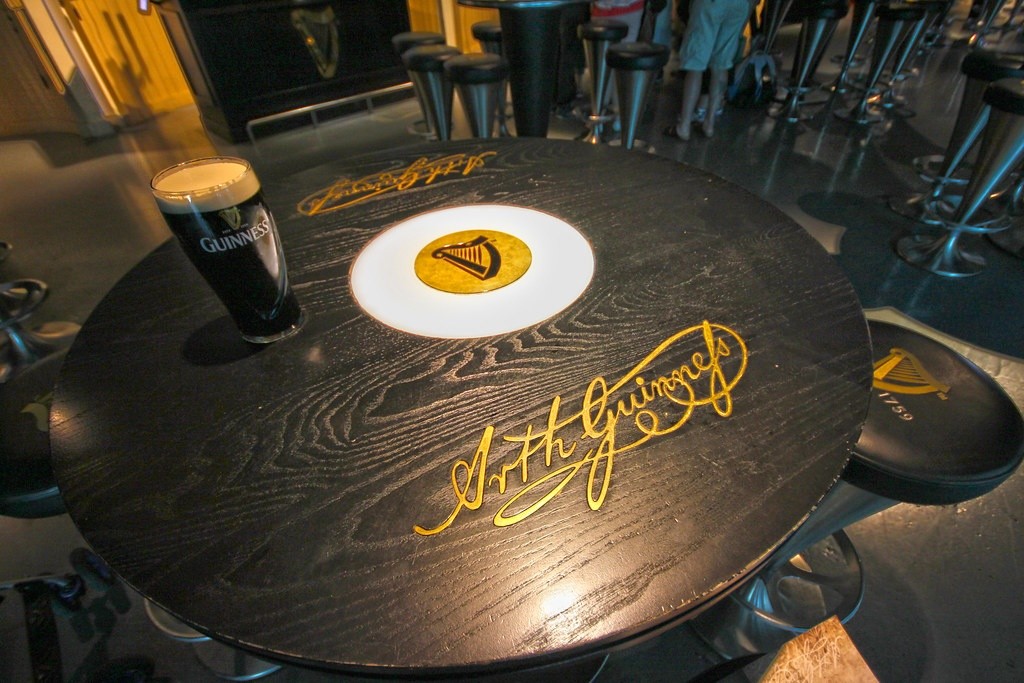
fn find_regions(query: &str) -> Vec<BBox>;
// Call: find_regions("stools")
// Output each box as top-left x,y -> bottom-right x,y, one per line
393,20 -> 670,156
745,1 -> 1024,277
0,243 -> 282,683
687,320 -> 1023,668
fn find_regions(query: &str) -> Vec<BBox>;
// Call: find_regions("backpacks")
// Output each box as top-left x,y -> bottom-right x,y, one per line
729,50 -> 777,106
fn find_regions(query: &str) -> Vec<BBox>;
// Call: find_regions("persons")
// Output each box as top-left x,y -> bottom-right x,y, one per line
661,0 -> 750,141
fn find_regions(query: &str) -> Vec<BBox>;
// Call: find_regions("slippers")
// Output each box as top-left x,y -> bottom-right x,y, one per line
665,126 -> 690,142
699,122 -> 713,139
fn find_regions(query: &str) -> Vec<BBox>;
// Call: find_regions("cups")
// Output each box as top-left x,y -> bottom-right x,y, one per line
149,154 -> 305,344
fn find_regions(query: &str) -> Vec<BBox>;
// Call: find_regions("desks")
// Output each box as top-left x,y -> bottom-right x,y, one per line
456,0 -> 584,138
49,138 -> 873,683
